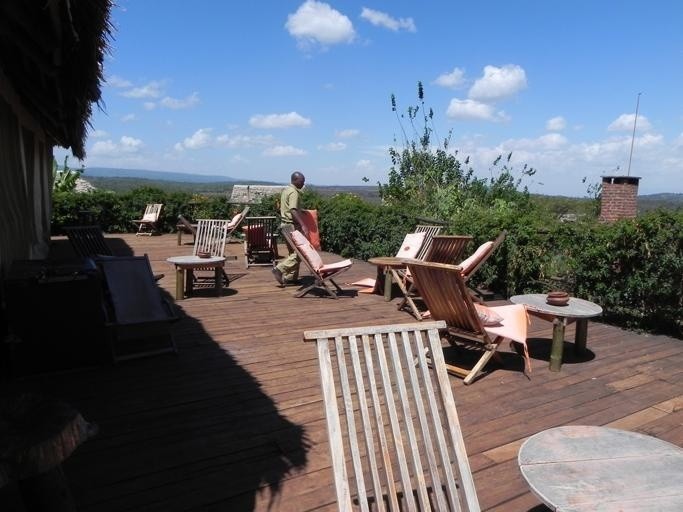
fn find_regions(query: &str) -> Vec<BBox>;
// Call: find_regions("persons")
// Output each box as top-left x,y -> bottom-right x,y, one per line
271,171 -> 311,288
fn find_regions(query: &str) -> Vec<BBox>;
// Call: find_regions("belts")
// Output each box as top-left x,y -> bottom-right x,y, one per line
280,220 -> 294,224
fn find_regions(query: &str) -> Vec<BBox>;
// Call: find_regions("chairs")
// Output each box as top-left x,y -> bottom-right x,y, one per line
281,229 -> 352,299
244,217 -> 276,267
131,204 -> 164,236
66,226 -> 110,258
192,219 -> 230,286
392,235 -> 505,318
303,321 -> 483,512
407,260 -> 525,386
516,425 -> 682,512
179,205 -> 250,247
92,254 -> 181,363
397,236 -> 472,320
395,225 -> 444,260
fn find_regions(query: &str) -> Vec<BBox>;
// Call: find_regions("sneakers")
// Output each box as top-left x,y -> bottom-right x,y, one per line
272,267 -> 283,284
284,278 -> 296,284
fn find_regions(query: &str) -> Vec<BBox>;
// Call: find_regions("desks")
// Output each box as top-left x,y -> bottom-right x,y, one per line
512,294 -> 602,372
369,257 -> 407,301
1,395 -> 89,510
168,255 -> 225,301
